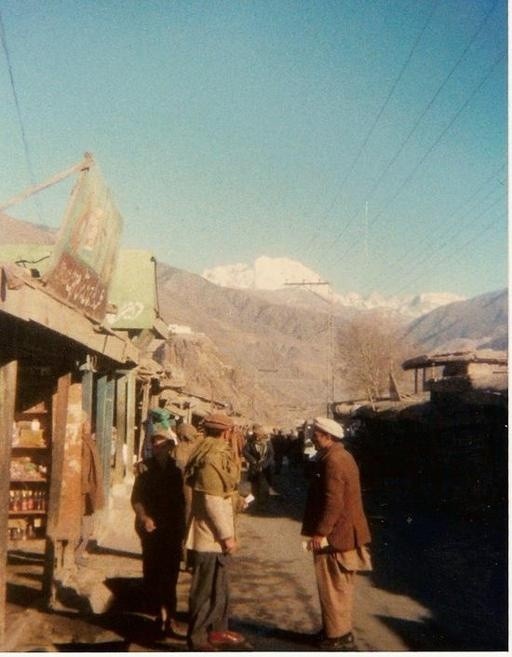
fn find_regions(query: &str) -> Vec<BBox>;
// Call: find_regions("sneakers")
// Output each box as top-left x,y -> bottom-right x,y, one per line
152,618 -> 188,641
193,628 -> 247,651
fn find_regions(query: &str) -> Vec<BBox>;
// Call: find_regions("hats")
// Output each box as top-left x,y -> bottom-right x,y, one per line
313,415 -> 345,440
203,413 -> 234,431
149,429 -> 178,446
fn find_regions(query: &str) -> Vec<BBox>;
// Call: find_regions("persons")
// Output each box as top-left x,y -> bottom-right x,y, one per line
130,427 -> 188,643
181,412 -> 249,650
299,414 -> 375,650
173,412 -> 305,573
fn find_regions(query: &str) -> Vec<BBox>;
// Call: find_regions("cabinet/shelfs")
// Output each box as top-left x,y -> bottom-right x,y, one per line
7,357 -> 55,552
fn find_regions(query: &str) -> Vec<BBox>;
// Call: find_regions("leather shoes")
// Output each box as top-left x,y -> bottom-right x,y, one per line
308,629 -> 356,650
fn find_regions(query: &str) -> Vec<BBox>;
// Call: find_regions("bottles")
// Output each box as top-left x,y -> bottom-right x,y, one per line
7,489 -> 49,542
